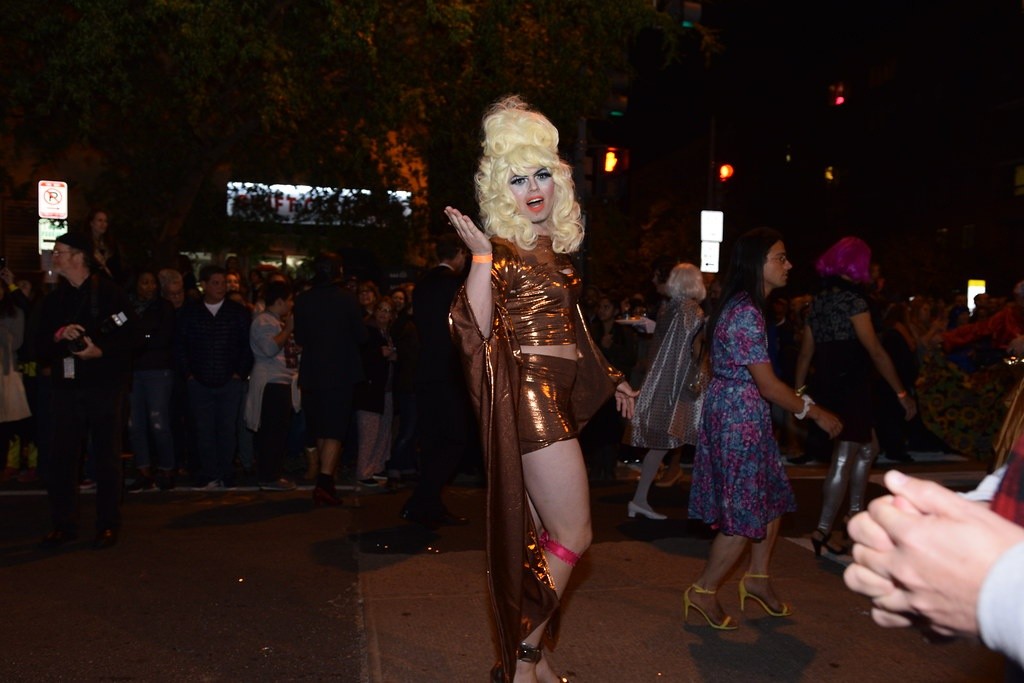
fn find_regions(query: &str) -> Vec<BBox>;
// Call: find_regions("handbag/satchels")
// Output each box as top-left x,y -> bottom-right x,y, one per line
0,334 -> 32,423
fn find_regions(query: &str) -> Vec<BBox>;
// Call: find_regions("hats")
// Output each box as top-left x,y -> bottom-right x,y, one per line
55,231 -> 95,257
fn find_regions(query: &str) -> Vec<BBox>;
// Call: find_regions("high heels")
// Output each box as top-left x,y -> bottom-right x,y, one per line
842,514 -> 852,539
738,571 -> 793,616
490,658 -> 577,683
627,501 -> 668,520
810,529 -> 849,557
655,465 -> 683,487
683,583 -> 738,630
312,487 -> 343,506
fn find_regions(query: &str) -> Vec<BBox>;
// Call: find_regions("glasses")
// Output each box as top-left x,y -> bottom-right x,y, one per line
374,306 -> 392,313
53,251 -> 74,256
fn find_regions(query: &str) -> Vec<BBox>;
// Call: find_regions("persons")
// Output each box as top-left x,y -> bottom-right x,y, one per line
795,238 -> 916,559
0,206 -> 471,489
287,250 -> 390,512
845,377 -> 1024,668
682,229 -> 844,630
27,231 -> 147,547
400,231 -> 472,527
578,265 -> 818,479
870,264 -> 1023,465
626,263 -> 710,522
444,95 -> 641,683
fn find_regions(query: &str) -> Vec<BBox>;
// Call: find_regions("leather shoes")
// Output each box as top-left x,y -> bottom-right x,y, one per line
39,527 -> 78,551
92,525 -> 118,548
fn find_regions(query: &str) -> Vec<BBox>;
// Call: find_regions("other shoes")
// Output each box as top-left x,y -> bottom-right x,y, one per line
357,478 -> 379,487
0,465 -> 316,495
372,471 -> 389,480
786,454 -> 815,464
884,448 -> 916,463
399,496 -> 469,525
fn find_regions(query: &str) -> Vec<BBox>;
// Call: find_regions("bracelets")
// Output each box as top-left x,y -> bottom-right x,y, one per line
898,391 -> 907,398
472,254 -> 493,263
55,327 -> 64,340
794,395 -> 815,420
